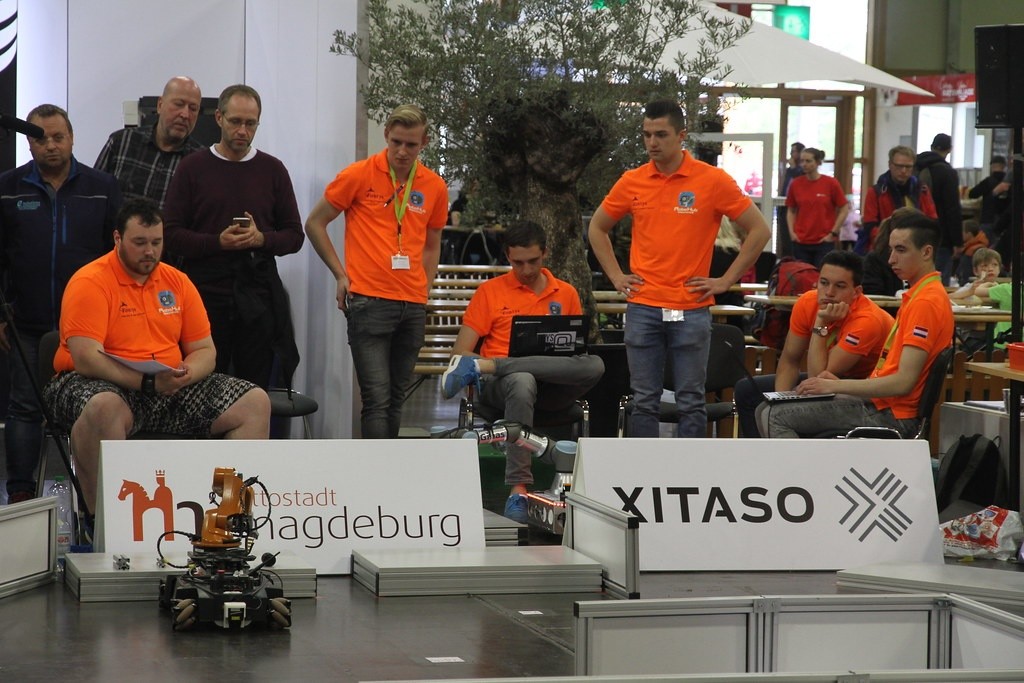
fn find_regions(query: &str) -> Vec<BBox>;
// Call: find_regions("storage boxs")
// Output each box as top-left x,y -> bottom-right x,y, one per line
1006,342 -> 1024,371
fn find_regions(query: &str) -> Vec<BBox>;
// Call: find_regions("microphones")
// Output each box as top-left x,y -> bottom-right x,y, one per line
0,114 -> 44,139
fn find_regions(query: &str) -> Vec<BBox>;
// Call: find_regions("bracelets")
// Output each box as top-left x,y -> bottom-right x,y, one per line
829,230 -> 838,237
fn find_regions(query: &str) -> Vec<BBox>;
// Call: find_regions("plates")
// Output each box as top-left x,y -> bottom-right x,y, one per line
972,305 -> 992,308
951,305 -> 967,308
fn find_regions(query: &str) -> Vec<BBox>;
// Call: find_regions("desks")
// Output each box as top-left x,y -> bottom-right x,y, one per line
443,226 -> 504,234
969,362 -> 1024,512
593,282 -> 1012,363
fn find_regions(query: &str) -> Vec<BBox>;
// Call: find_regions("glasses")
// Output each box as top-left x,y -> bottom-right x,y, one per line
29,133 -> 69,145
222,111 -> 260,128
892,162 -> 916,170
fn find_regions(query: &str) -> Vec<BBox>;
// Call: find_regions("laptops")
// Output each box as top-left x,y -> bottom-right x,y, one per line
723,341 -> 835,404
508,314 -> 591,357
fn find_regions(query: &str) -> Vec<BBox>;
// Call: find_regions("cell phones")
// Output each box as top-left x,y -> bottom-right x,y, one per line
232,218 -> 250,228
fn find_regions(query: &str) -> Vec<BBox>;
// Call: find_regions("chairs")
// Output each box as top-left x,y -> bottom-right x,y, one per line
458,337 -> 589,437
267,372 -> 318,439
618,322 -> 746,437
835,346 -> 954,440
33,330 -> 83,542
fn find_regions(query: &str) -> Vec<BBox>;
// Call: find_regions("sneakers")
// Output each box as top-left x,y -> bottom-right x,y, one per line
504,493 -> 528,524
441,354 -> 482,401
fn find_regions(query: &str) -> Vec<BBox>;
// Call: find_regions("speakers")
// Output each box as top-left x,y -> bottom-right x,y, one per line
975,24 -> 1024,128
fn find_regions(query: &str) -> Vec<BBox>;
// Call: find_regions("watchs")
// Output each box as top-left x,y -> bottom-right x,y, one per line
812,327 -> 828,337
141,373 -> 155,397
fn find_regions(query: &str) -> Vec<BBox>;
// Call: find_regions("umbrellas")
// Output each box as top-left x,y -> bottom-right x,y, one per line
373,0 -> 936,97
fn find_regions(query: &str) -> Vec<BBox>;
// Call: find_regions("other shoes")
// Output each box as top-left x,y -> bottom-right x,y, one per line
85,515 -> 95,543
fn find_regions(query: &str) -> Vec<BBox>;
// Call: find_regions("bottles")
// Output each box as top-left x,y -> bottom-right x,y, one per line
48,476 -> 71,581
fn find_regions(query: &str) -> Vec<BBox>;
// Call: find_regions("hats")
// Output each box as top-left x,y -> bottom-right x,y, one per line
990,156 -> 1007,165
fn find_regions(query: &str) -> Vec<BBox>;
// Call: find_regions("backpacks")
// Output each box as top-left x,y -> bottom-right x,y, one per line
935,434 -> 1008,514
911,162 -> 948,195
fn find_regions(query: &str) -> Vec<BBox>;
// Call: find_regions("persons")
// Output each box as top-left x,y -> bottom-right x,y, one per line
162,85 -> 305,395
94,76 -> 206,207
734,249 -> 895,438
754,212 -> 954,438
42,200 -> 271,544
305,105 -> 449,439
588,99 -> 772,438
441,220 -> 605,524
710,133 -> 1024,354
0,104 -> 124,505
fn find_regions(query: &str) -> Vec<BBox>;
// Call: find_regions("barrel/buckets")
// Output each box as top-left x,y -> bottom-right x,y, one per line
1006,341 -> 1024,372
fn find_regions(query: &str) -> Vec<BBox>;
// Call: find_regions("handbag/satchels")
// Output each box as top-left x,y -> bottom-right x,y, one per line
853,221 -> 879,255
